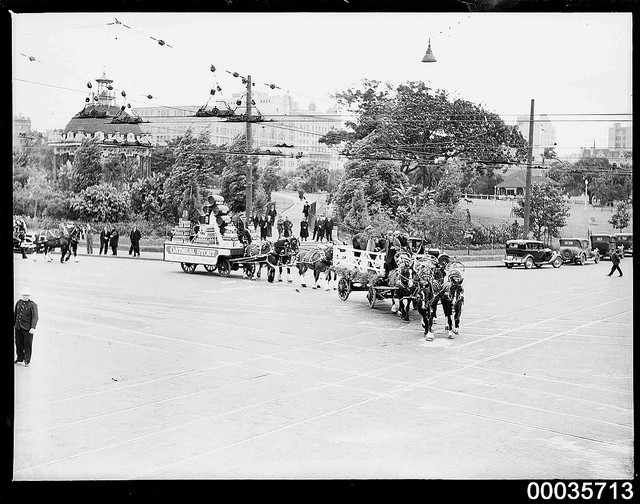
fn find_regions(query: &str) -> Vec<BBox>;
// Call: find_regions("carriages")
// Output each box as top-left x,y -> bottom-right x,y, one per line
13,222 -> 98,264
163,236 -> 349,291
329,243 -> 464,341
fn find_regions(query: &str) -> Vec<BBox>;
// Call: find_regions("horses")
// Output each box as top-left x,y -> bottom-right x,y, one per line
36,224 -> 79,263
295,242 -> 338,288
441,276 -> 466,340
257,237 -> 301,281
320,242 -> 347,291
387,264 -> 418,323
413,276 -> 442,341
424,265 -> 446,324
244,238 -> 290,282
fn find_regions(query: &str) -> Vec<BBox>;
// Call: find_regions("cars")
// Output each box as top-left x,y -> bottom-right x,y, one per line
407,238 -> 439,259
502,240 -> 563,269
554,238 -> 600,265
590,235 -> 625,260
615,233 -> 633,257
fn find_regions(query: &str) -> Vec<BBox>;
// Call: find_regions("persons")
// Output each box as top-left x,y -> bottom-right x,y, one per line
254,204 -> 277,241
283,216 -> 293,237
353,225 -> 407,253
302,201 -> 310,219
130,225 -> 141,256
129,245 -> 134,255
300,218 -> 309,241
14,288 -> 39,366
277,216 -> 284,238
312,216 -> 320,240
512,220 -> 519,239
316,216 -> 325,242
99,226 -> 110,255
85,224 -> 97,254
326,217 -> 333,241
607,247 -> 623,277
110,227 -> 119,255
